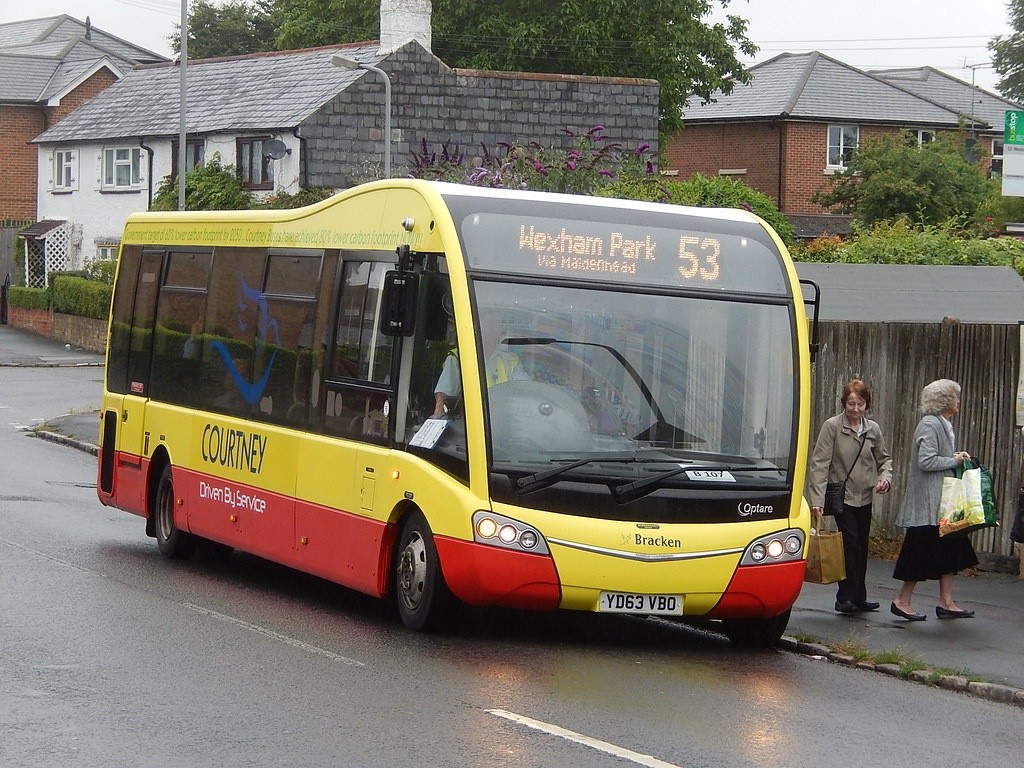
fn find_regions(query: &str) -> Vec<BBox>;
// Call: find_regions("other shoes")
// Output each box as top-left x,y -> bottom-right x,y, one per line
936,605 -> 974,619
834,600 -> 859,613
860,600 -> 880,611
891,601 -> 927,621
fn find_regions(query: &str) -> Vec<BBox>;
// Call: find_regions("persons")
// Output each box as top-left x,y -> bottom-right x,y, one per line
431,311 -> 530,417
890,379 -> 980,621
808,379 -> 893,612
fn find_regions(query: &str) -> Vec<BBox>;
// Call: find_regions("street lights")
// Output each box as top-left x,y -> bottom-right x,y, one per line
332,55 -> 392,178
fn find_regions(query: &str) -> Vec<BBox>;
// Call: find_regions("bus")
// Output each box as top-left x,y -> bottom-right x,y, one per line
98,179 -> 820,644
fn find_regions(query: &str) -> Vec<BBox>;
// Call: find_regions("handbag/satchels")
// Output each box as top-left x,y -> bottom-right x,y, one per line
939,456 -> 1000,538
823,481 -> 845,515
804,512 -> 846,585
